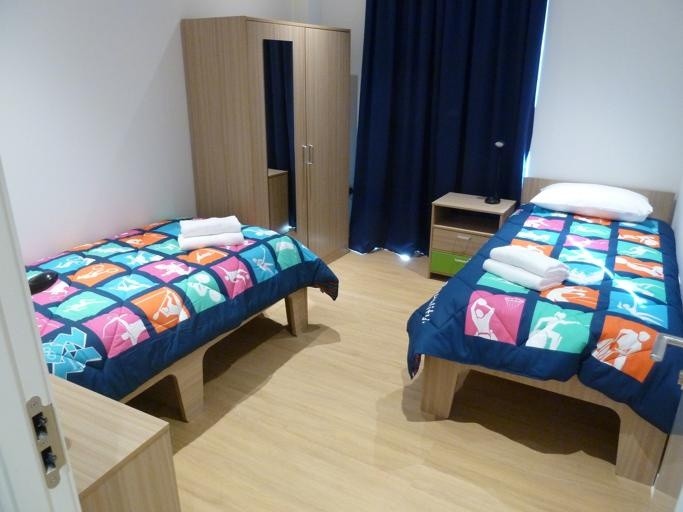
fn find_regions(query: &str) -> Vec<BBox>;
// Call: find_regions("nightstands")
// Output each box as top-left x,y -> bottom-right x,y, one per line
426,191 -> 516,281
42,375 -> 185,512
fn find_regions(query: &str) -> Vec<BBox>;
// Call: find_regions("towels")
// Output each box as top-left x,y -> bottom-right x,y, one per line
176,231 -> 244,249
489,244 -> 569,282
180,214 -> 241,236
481,258 -> 568,291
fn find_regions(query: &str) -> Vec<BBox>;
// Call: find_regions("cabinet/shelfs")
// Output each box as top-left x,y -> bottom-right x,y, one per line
179,17 -> 351,263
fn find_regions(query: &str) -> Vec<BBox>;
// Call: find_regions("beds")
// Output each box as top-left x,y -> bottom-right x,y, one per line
21,218 -> 339,423
407,177 -> 683,486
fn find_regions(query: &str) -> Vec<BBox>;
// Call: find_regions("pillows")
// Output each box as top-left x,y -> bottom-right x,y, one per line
529,182 -> 653,222
